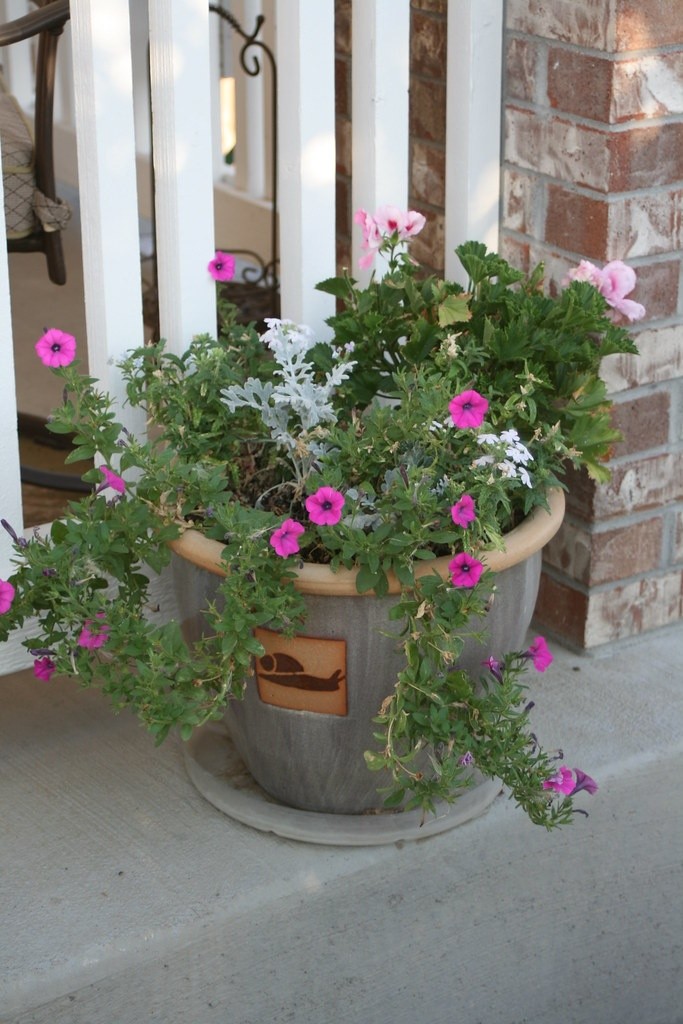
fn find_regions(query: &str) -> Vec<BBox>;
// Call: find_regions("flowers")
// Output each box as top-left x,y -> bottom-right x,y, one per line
0,204 -> 649,833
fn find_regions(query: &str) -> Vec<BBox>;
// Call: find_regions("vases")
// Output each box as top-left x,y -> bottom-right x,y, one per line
151,455 -> 566,848
